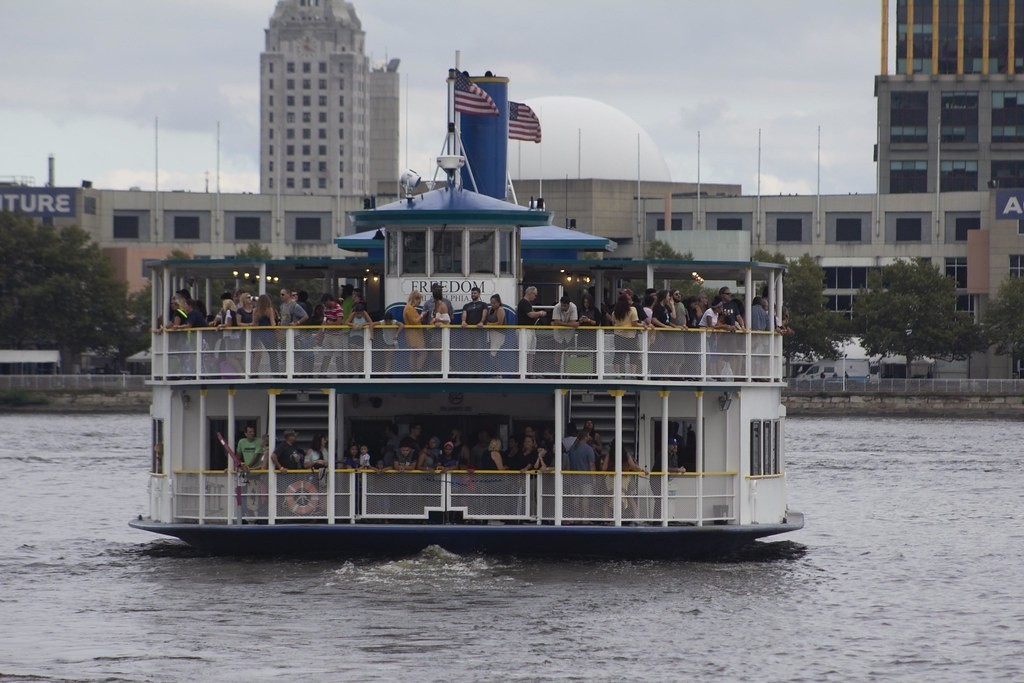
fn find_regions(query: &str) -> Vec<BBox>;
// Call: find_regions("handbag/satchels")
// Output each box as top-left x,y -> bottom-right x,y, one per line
311,464 -> 326,481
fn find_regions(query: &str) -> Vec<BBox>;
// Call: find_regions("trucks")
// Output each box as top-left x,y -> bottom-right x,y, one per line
796,356 -> 871,389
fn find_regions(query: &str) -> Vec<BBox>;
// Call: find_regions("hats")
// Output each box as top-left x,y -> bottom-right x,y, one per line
444,440 -> 454,449
283,428 -> 298,440
668,438 -> 679,445
341,284 -> 353,292
432,283 -> 445,291
619,289 -> 633,297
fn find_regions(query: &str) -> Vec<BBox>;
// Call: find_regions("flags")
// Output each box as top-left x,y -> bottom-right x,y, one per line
508,100 -> 542,144
453,68 -> 499,116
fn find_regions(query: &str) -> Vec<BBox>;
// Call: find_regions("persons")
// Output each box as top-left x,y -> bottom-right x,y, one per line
155,279 -> 796,383
224,408 -> 688,526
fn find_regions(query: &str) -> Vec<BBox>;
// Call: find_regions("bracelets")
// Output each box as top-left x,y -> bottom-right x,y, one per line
588,319 -> 592,323
280,467 -> 284,470
249,322 -> 250,325
427,466 -> 428,470
672,324 -> 675,326
742,325 -> 745,328
297,321 -> 302,325
643,323 -> 647,326
221,324 -> 223,326
639,468 -> 643,472
480,320 -> 484,323
547,467 -> 549,471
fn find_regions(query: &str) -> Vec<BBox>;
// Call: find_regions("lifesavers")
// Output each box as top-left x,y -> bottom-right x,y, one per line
285,480 -> 320,515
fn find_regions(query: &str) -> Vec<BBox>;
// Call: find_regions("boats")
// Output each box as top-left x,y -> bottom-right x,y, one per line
122,45 -> 803,554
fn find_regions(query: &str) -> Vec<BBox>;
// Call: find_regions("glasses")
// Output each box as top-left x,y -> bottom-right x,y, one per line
473,303 -> 476,310
723,293 -> 731,295
292,294 -> 299,296
673,292 -> 681,296
279,294 -> 287,296
533,293 -> 537,298
